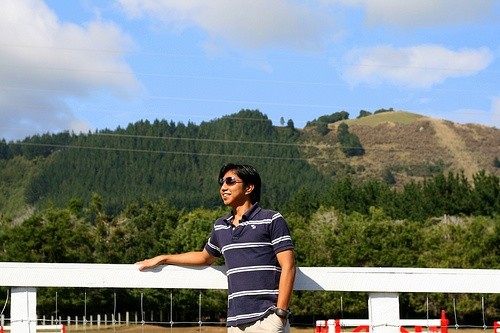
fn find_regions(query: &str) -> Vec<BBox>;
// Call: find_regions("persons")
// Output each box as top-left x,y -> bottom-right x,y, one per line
137,163 -> 296,332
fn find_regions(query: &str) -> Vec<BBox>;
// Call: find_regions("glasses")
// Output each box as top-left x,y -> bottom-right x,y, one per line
219,177 -> 243,185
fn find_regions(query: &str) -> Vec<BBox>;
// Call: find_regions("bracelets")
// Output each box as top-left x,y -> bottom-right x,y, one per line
273,308 -> 291,319
